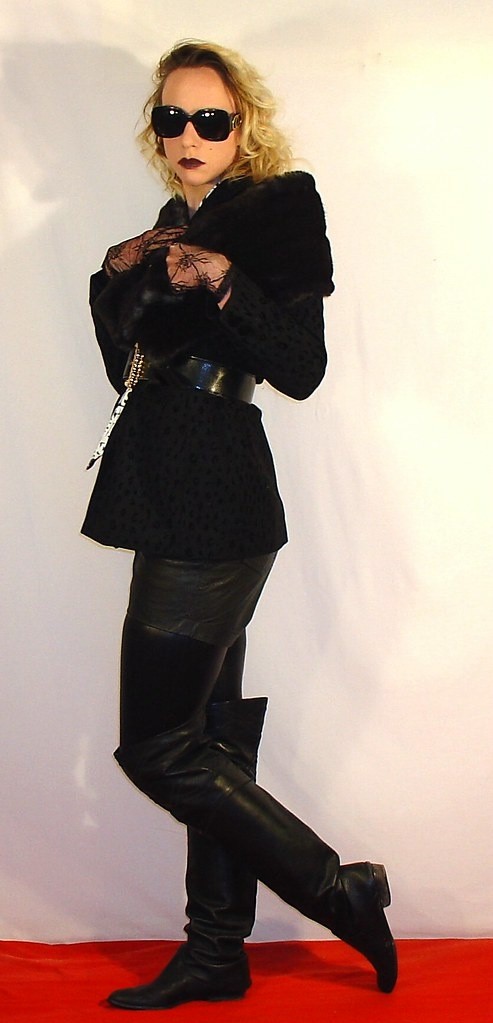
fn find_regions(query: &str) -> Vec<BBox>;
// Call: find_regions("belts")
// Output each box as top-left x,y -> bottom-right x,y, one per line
119,346 -> 256,406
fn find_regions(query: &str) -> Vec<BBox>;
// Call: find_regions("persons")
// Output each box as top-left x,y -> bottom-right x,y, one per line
79,39 -> 398,1011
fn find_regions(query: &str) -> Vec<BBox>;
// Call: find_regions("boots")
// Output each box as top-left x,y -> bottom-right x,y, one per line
113,710 -> 397,994
107,698 -> 267,1010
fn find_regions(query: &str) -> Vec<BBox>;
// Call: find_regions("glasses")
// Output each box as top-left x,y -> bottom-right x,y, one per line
151,105 -> 241,142
